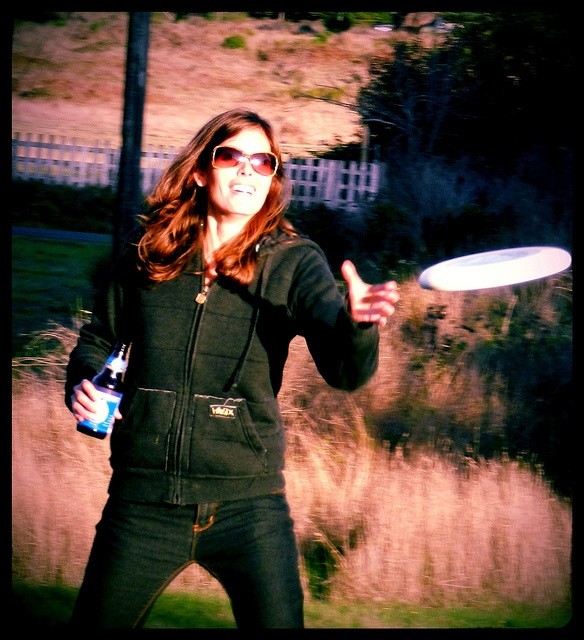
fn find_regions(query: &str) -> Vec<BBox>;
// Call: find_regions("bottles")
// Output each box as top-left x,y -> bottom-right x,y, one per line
77,343 -> 130,441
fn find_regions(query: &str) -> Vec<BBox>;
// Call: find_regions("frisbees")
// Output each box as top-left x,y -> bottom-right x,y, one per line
419,247 -> 572,292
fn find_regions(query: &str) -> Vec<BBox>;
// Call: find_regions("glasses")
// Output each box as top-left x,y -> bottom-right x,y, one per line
212,146 -> 279,177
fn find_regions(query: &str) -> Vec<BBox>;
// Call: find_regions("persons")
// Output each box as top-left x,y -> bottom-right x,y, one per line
64,104 -> 398,628
